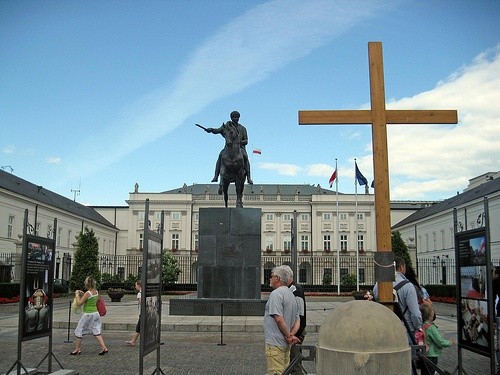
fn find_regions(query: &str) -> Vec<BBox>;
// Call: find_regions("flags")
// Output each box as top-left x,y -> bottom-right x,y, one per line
253,146 -> 261,155
328,169 -> 336,188
355,162 -> 368,186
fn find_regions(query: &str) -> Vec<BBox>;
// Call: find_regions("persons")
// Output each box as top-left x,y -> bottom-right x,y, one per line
492,266 -> 500,372
124,280 -> 146,347
371,254 -> 424,375
281,265 -> 308,374
403,263 -> 434,323
263,265 -> 302,375
461,302 -> 489,346
361,289 -> 373,301
206,111 -> 254,185
71,276 -> 109,357
466,268 -> 485,299
416,302 -> 459,375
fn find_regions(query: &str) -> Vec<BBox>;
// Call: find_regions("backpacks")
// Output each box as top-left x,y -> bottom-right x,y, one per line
415,322 -> 433,353
392,279 -> 408,321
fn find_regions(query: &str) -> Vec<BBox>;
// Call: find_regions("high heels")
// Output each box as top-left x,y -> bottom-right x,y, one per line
69,349 -> 81,356
99,348 -> 108,355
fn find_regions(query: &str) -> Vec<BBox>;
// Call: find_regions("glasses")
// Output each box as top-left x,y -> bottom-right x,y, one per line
270,275 -> 277,279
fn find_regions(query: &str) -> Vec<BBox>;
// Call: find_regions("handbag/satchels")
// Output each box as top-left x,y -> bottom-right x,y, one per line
96,289 -> 107,316
